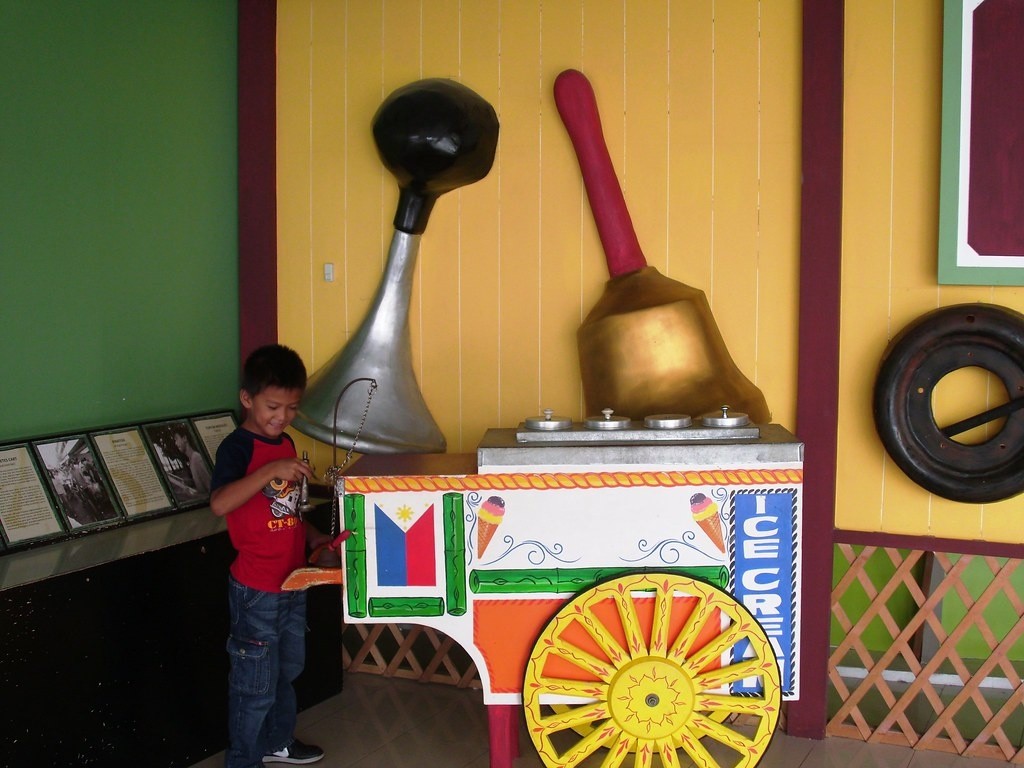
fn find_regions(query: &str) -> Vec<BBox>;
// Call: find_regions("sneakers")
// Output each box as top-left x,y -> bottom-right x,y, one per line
261,738 -> 324,764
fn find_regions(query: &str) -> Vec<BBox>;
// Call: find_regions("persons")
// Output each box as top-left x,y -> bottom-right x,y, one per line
208,343 -> 341,768
49,458 -> 117,525
171,425 -> 212,495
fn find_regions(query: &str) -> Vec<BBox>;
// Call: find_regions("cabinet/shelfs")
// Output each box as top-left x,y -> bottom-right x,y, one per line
0,483 -> 343,768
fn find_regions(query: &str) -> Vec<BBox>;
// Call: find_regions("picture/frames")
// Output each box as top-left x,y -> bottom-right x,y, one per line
0,408 -> 242,554
